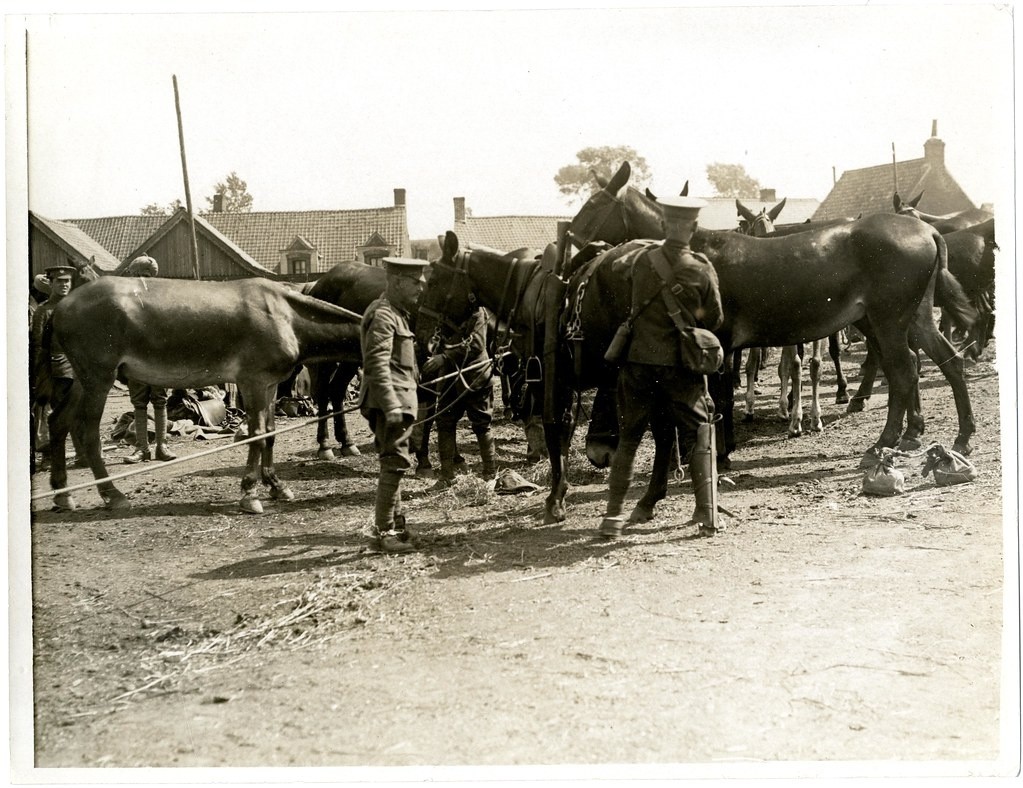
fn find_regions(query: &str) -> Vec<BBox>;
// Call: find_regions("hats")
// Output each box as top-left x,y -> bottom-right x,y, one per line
129,256 -> 158,277
34,274 -> 53,294
656,196 -> 708,219
44,266 -> 77,279
382,256 -> 430,282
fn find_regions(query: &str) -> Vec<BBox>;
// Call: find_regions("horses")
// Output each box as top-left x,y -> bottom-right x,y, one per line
29,158 -> 997,535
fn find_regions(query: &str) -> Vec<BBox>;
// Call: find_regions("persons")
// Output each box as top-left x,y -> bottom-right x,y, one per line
422,308 -> 497,491
360,257 -> 431,551
600,197 -> 724,538
124,256 -> 176,465
31,266 -> 106,472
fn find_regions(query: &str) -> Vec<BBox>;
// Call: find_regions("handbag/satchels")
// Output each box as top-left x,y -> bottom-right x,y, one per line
682,326 -> 723,375
603,321 -> 633,361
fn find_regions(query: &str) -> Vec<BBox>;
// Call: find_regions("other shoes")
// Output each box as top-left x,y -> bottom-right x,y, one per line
80,459 -> 106,469
698,521 -> 726,537
155,445 -> 177,461
396,529 -> 421,544
379,536 -> 416,554
599,517 -> 623,536
40,456 -> 52,471
425,478 -> 458,492
123,449 -> 151,463
